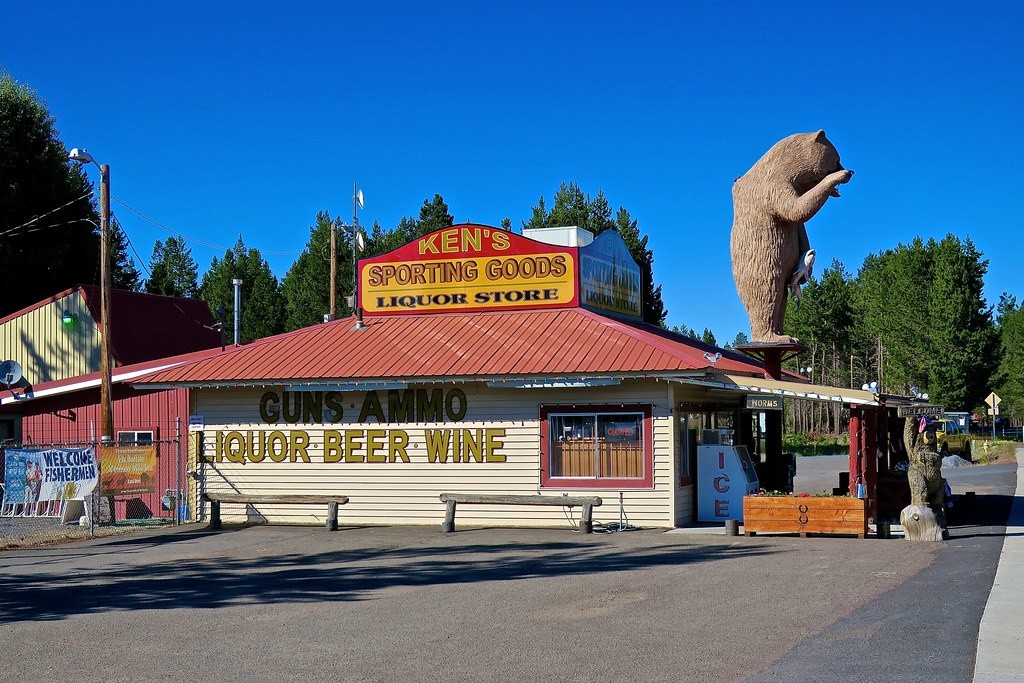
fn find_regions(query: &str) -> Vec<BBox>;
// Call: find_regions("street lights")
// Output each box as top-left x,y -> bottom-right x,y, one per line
68,146 -> 115,450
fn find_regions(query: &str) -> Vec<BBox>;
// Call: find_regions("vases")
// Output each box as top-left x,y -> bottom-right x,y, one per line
743,495 -> 870,539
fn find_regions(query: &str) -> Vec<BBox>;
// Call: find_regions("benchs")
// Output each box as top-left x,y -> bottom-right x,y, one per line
439,492 -> 602,534
200,493 -> 350,533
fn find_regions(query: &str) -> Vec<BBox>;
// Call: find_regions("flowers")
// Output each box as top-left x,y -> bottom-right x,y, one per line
750,487 -> 851,496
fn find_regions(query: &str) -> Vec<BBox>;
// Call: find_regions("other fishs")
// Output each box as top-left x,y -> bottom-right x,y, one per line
787,249 -> 816,309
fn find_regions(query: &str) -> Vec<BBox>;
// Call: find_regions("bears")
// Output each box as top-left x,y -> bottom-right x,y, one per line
904,416 -> 948,512
730,129 -> 854,343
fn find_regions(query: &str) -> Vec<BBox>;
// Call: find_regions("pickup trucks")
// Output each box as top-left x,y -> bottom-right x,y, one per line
935,419 -> 973,459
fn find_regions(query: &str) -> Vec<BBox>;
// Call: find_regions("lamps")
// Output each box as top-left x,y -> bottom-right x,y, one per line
63,310 -> 72,324
800,366 -> 814,378
704,351 -> 722,367
